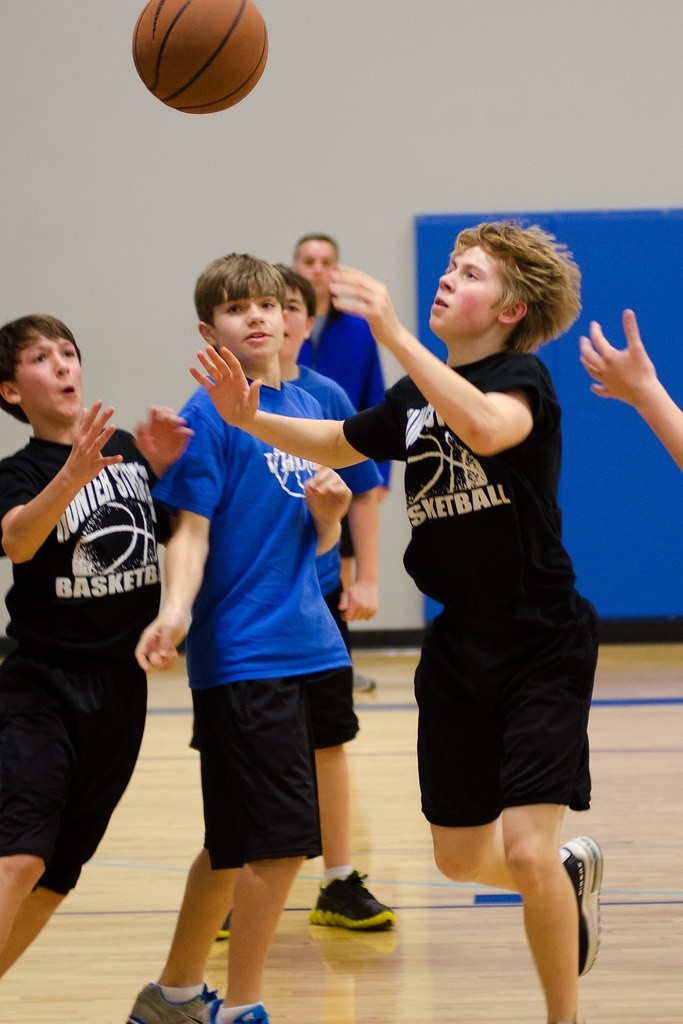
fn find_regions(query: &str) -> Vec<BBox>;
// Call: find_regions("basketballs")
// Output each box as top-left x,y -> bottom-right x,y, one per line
132,0 -> 269,115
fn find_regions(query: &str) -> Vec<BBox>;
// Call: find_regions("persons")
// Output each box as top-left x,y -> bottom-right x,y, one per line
1,314 -> 197,976
579,308 -> 683,470
190,219 -> 603,1024
215,264 -> 394,943
292,236 -> 393,589
126,250 -> 351,1024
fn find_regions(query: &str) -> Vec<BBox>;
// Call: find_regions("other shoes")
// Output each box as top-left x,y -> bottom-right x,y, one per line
354,674 -> 376,693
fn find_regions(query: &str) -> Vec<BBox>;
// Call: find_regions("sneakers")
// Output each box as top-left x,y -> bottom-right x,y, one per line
309,922 -> 395,964
128,983 -> 270,1024
558,836 -> 604,976
216,912 -> 232,938
312,871 -> 395,925
